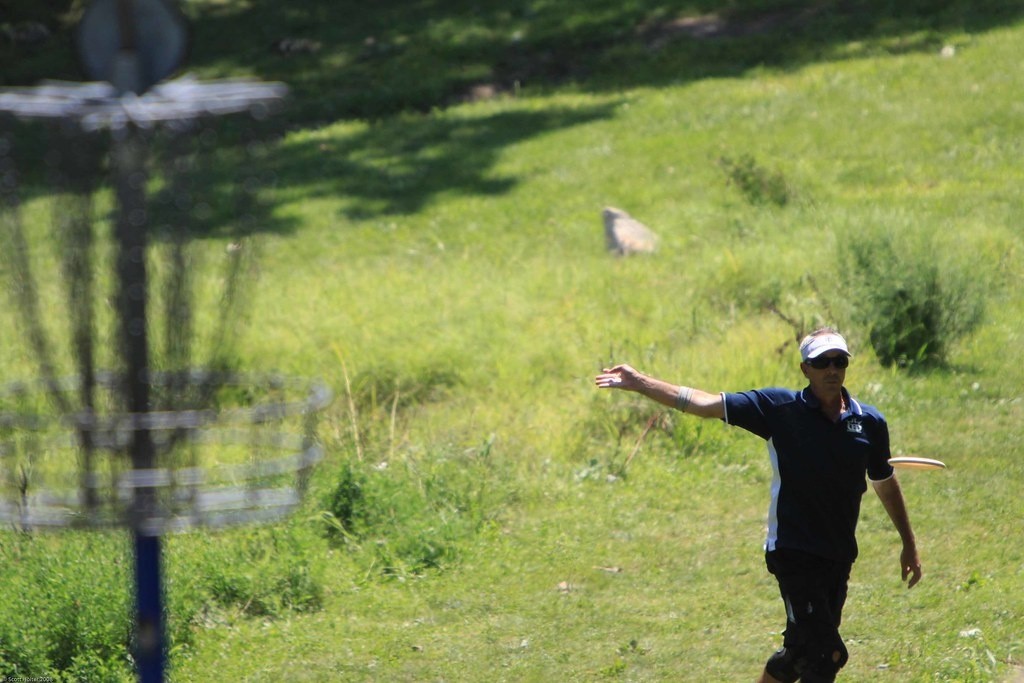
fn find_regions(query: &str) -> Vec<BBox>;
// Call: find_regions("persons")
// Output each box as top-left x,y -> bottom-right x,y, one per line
594,330 -> 925,683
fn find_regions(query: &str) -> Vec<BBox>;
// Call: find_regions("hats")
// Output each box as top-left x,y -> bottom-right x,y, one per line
800,331 -> 852,360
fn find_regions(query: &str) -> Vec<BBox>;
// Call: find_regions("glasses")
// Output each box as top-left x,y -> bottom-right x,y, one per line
804,354 -> 849,369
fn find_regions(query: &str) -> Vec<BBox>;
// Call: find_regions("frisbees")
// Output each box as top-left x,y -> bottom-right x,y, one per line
888,457 -> 946,471
